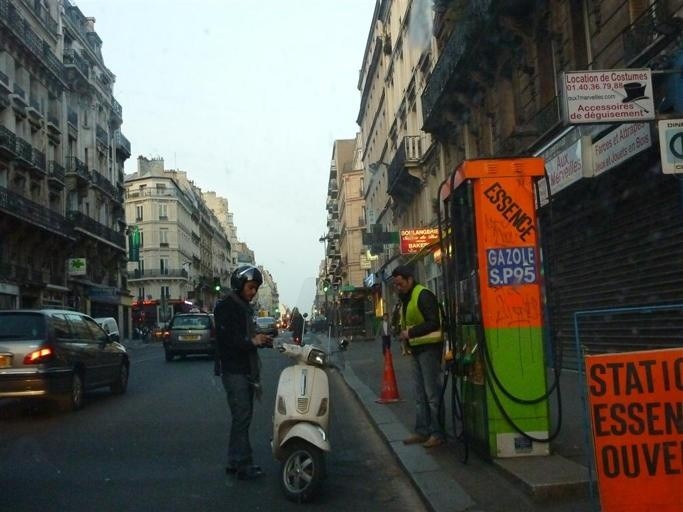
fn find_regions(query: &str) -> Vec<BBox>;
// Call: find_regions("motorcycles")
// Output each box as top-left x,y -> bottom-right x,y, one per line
270,335 -> 350,501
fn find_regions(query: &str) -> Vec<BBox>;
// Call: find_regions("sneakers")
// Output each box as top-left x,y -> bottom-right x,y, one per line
404,432 -> 444,448
225,463 -> 266,481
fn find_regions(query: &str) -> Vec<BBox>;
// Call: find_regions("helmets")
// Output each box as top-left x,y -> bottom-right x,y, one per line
230,265 -> 263,290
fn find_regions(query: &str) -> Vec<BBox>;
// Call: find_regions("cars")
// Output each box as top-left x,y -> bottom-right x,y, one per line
163,312 -> 215,361
0,308 -> 130,413
252,316 -> 278,337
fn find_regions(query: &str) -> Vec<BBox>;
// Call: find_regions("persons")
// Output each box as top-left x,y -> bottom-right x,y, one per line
209,264 -> 274,479
391,263 -> 450,448
288,307 -> 304,346
375,312 -> 393,356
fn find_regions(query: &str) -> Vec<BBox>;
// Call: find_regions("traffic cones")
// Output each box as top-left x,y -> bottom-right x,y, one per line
375,347 -> 402,404
